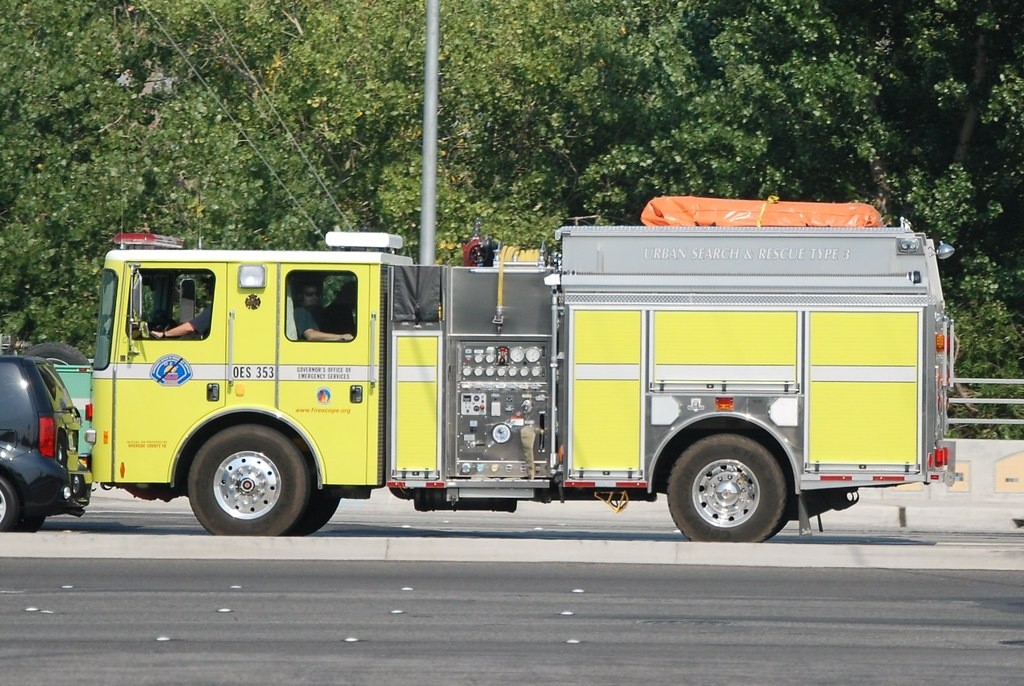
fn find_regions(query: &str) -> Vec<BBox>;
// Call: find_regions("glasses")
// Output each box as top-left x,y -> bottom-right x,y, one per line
303,291 -> 319,297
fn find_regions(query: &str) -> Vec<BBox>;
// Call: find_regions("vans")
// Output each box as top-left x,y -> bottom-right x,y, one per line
20,343 -> 92,467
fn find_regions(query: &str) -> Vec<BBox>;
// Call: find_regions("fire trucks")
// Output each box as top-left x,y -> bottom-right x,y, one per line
79,195 -> 960,538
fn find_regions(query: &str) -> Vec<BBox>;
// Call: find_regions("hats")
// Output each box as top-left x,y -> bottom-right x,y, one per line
200,276 -> 215,284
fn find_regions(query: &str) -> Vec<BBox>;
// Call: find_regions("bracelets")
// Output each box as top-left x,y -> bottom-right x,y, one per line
162,330 -> 165,337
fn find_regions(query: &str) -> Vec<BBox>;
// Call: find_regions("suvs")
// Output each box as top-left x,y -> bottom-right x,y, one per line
0,356 -> 87,533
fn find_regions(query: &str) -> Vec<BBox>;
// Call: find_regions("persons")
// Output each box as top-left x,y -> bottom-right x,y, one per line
293,277 -> 354,342
151,275 -> 216,339
